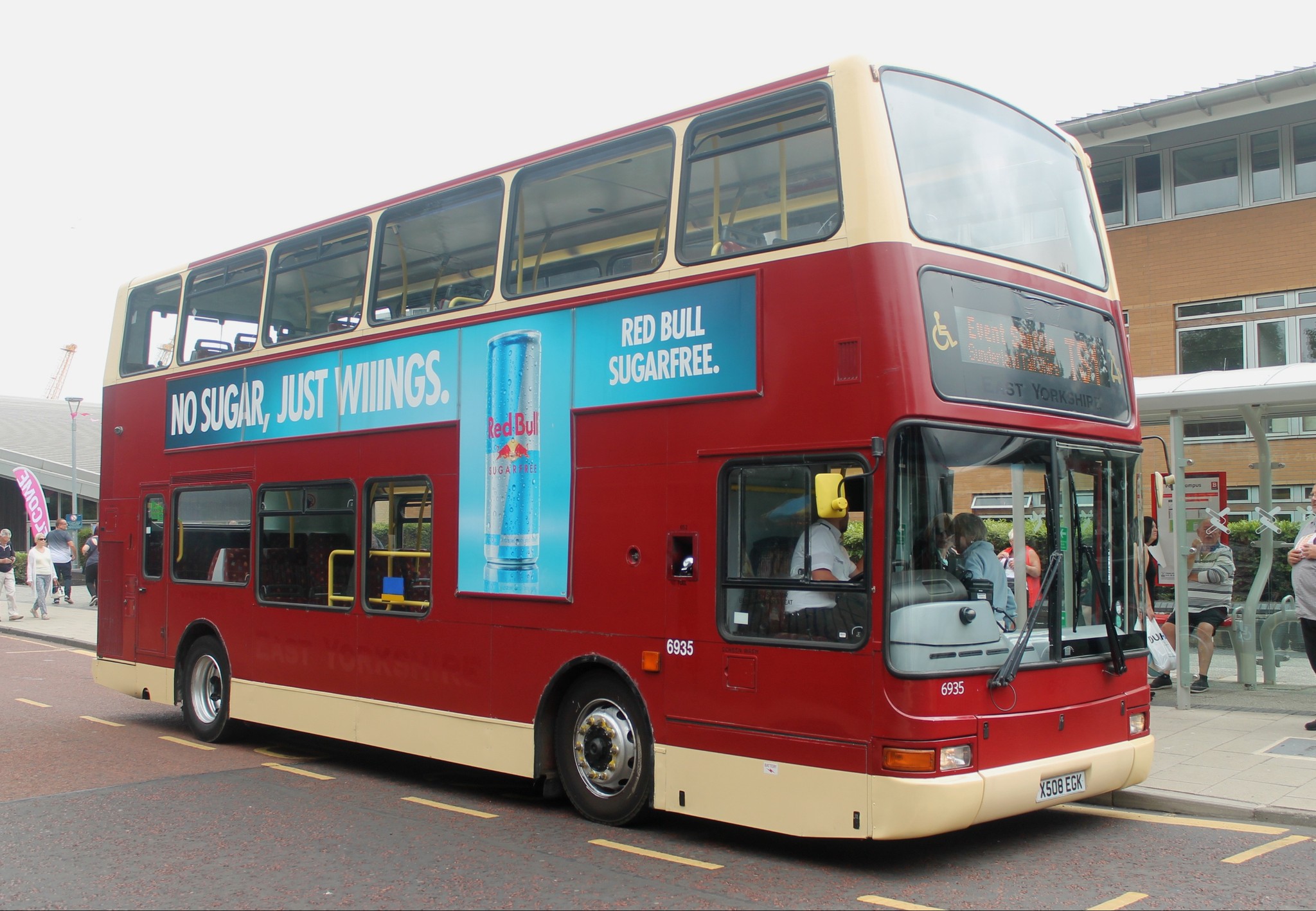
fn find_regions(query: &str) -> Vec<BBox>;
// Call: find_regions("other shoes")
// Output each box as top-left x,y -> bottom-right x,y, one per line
9,615 -> 24,620
1305,719 -> 1316,731
55,600 -> 59,604
42,615 -> 50,620
65,595 -> 73,604
90,596 -> 98,606
30,609 -> 39,617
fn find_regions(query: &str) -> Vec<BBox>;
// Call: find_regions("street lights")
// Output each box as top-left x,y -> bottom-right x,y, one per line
65,397 -> 83,571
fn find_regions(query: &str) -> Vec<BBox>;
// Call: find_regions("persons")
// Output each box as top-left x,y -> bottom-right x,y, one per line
0,529 -> 24,622
914,512 -> 958,575
26,533 -> 57,619
784,500 -> 864,642
82,526 -> 98,606
44,519 -> 77,604
1288,485 -> 1316,731
1133,516 -> 1158,621
949,512 -> 1018,629
998,530 -> 1042,629
1082,571 -> 1093,625
1150,519 -> 1235,692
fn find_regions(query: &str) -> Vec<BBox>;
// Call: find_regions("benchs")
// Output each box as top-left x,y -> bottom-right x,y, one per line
1155,605 -> 1245,685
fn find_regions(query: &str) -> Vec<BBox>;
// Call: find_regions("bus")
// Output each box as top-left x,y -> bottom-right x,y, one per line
95,55 -> 1176,844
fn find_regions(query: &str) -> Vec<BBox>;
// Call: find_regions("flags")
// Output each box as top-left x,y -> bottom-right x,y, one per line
15,469 -> 49,545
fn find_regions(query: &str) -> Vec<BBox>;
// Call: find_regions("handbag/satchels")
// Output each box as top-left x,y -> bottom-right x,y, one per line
51,581 -> 64,598
1146,616 -> 1177,673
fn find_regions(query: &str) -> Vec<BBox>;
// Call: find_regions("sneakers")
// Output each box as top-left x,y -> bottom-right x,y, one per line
1189,678 -> 1209,693
1149,677 -> 1173,690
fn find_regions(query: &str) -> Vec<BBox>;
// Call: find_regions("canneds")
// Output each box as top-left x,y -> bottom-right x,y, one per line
483,563 -> 539,595
484,329 -> 542,565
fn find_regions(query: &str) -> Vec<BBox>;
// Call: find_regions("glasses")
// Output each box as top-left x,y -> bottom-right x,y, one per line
1308,493 -> 1316,499
60,523 -> 67,525
38,539 -> 46,541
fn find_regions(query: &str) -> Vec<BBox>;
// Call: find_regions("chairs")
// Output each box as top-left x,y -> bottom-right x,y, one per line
143,532 -> 432,614
126,283 -> 490,376
750,536 -> 836,643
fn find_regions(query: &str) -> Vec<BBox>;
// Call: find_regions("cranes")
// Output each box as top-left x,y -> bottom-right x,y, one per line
45,343 -> 78,399
153,343 -> 174,367
